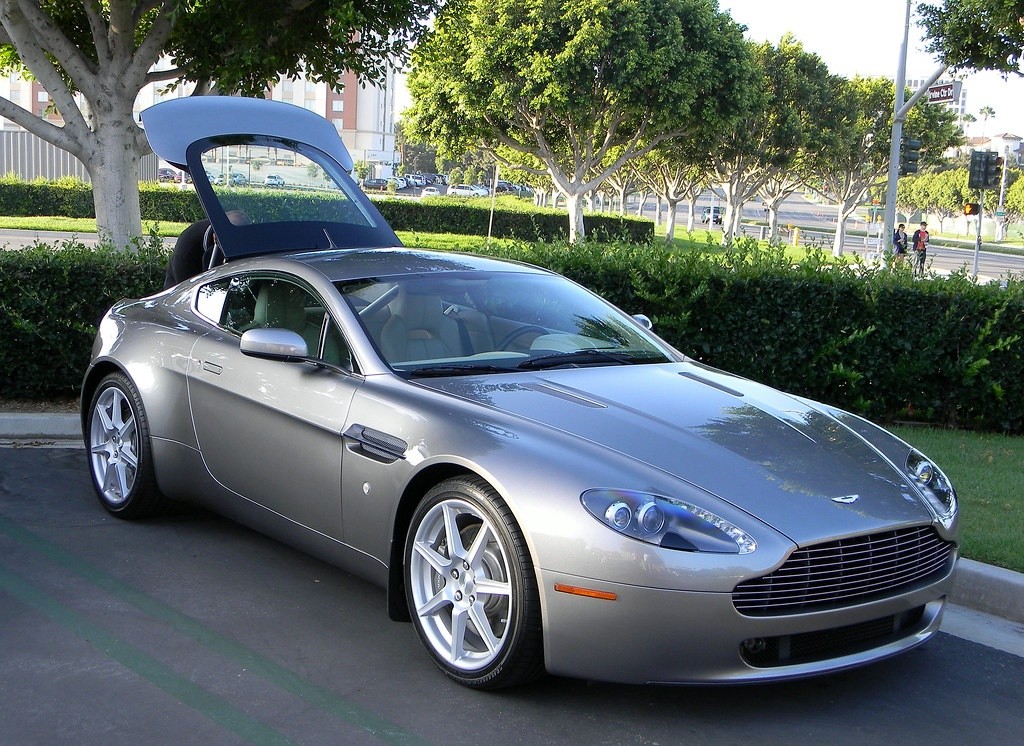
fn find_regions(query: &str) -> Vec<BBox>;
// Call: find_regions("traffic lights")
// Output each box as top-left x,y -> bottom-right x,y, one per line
964,203 -> 980,215
982,154 -> 1005,188
900,137 -> 922,175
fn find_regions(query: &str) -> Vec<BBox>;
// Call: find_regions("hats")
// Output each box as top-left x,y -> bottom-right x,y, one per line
898,223 -> 906,228
920,221 -> 928,226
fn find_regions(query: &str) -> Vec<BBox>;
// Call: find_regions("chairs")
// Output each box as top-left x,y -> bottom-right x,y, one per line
238,282 -> 350,369
379,286 -> 462,364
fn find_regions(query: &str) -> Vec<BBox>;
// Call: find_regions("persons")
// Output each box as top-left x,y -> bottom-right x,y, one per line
162,209 -> 252,290
893,223 -> 907,262
912,221 -> 929,273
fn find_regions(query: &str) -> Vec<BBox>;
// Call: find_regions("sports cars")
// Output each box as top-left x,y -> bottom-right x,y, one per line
80,94 -> 962,693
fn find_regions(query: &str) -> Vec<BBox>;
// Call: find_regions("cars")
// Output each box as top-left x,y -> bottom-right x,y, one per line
358,172 -> 536,198
158,167 -> 176,182
205,171 -> 249,188
176,170 -> 191,183
264,174 -> 286,187
421,187 -> 440,198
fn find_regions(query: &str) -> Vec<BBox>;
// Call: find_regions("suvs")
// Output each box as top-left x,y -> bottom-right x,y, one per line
700,205 -> 723,224
